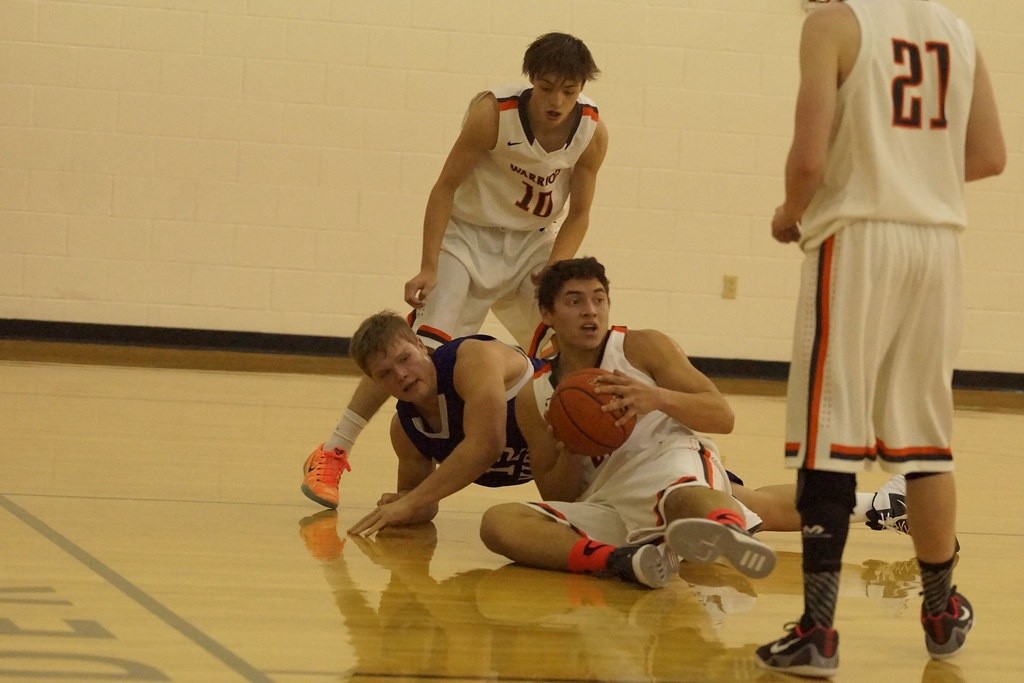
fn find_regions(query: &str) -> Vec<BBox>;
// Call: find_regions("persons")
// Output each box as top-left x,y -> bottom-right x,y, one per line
299,31 -> 609,512
347,309 -> 962,553
754,1 -> 1009,676
478,256 -> 777,590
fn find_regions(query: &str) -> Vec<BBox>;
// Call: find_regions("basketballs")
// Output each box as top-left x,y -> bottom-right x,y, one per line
548,367 -> 638,457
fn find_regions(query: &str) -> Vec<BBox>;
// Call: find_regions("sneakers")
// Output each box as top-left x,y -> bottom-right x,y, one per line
299,507 -> 343,556
753,622 -> 839,677
607,543 -> 679,590
873,475 -> 961,552
301,443 -> 351,508
862,558 -> 919,602
920,585 -> 973,656
665,517 -> 776,580
